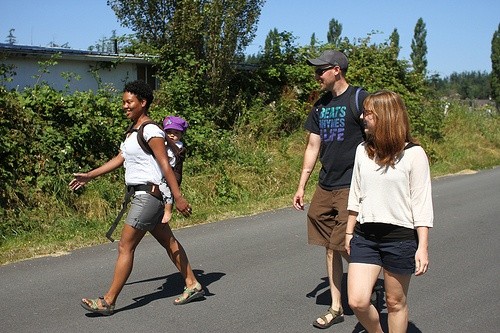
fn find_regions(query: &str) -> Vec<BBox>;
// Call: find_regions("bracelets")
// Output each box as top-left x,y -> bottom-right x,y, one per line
345,232 -> 354,235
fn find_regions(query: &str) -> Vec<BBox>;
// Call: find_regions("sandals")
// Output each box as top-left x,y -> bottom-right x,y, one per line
313,307 -> 344,328
371,287 -> 378,308
80,296 -> 115,315
174,282 -> 205,305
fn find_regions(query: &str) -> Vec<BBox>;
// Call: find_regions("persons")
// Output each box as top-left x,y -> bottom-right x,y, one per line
69,80 -> 205,315
159,116 -> 188,223
344,91 -> 434,333
293,50 -> 381,329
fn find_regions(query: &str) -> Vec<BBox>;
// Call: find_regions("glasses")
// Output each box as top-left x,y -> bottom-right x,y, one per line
315,66 -> 343,76
361,110 -> 378,118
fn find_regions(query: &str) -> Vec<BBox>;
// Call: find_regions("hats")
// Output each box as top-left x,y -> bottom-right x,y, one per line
306,50 -> 348,69
163,116 -> 189,133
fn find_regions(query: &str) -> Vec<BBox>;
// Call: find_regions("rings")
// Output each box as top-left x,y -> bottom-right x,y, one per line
188,209 -> 190,211
